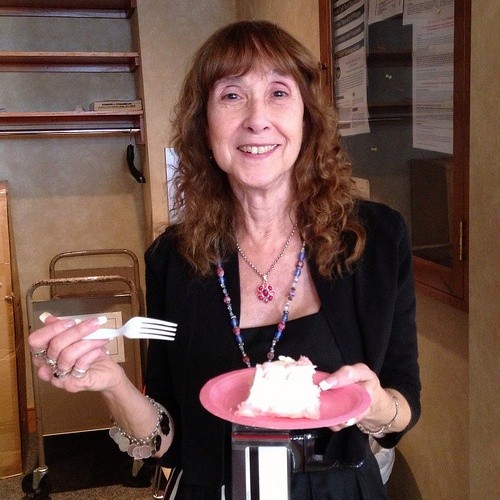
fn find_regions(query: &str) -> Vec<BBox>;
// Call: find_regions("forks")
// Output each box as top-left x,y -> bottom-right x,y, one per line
82,317 -> 178,341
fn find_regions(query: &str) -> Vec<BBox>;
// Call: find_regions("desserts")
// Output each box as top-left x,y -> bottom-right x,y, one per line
235,354 -> 320,420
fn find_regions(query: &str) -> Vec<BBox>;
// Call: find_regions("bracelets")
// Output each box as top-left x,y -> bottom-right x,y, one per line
356,388 -> 400,435
108,400 -> 171,460
113,394 -> 163,445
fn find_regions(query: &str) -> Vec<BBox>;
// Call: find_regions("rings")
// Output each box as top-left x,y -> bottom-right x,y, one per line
344,416 -> 357,429
51,366 -> 71,378
43,355 -> 57,368
70,367 -> 86,379
30,349 -> 49,360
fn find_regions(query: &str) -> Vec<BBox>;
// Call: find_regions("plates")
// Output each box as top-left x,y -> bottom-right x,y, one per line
199,368 -> 371,430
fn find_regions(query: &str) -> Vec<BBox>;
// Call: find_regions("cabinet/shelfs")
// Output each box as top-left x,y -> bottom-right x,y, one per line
0,0 -> 145,145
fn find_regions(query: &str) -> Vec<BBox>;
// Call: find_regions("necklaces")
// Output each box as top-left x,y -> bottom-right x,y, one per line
214,235 -> 308,369
234,214 -> 300,304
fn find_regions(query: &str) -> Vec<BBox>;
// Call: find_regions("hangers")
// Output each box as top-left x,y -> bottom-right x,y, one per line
127,127 -> 146,184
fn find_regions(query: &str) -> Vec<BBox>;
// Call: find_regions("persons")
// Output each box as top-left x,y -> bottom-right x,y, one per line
26,19 -> 423,500
